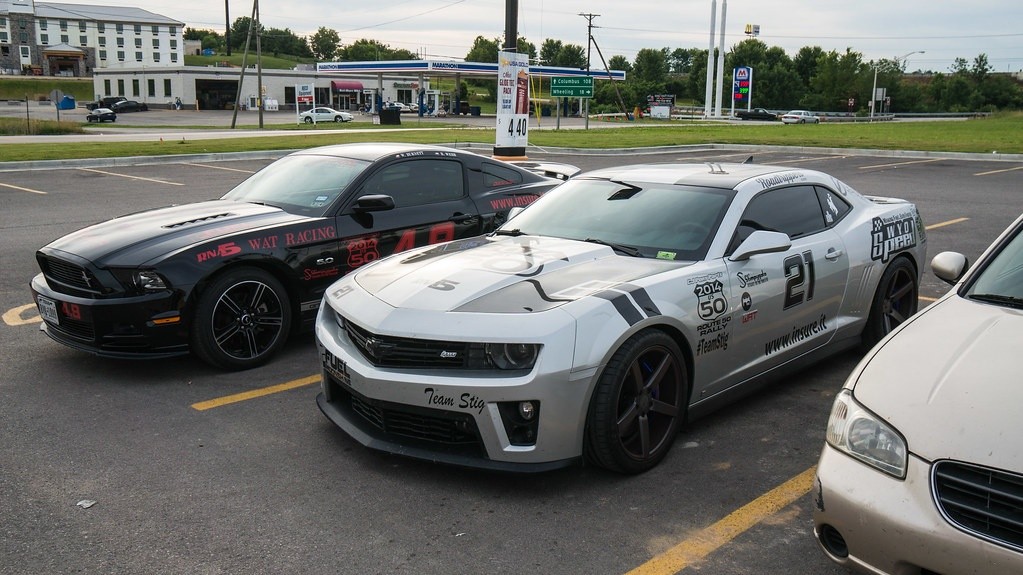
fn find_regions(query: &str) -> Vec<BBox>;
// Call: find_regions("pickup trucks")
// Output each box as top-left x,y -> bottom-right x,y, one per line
736,108 -> 778,121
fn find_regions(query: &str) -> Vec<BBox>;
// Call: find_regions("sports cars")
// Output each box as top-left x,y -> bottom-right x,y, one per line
314,156 -> 927,480
31,144 -> 585,375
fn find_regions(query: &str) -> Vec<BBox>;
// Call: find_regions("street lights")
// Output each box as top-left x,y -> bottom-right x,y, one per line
870,51 -> 926,122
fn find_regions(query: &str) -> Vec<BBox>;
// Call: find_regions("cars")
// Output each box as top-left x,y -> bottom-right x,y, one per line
86,109 -> 117,123
406,102 -> 419,113
111,101 -> 149,114
393,103 -> 410,113
810,212 -> 1023,574
782,110 -> 820,124
298,107 -> 355,123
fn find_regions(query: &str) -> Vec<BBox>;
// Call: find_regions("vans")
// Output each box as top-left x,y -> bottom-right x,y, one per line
101,97 -> 128,108
450,101 -> 470,115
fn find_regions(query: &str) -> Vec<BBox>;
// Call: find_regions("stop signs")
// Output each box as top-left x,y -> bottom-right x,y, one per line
848,99 -> 854,106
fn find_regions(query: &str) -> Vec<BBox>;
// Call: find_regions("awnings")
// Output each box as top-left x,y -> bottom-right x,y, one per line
331,80 -> 363,93
